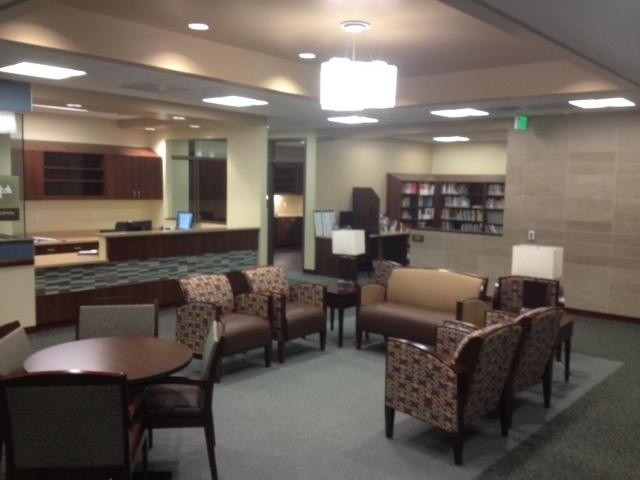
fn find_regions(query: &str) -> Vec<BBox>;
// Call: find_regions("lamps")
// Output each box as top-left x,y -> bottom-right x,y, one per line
332,229 -> 366,291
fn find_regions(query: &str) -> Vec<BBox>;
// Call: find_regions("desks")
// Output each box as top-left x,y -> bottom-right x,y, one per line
315,186 -> 409,280
24,336 -> 193,472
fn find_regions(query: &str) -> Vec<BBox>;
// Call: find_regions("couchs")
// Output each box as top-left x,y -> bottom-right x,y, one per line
178,273 -> 271,382
443,306 -> 565,408
494,276 -> 562,363
356,266 -> 489,351
386,323 -> 522,466
241,266 -> 327,364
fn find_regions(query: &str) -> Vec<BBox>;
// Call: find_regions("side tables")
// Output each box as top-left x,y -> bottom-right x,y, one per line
325,282 -> 369,348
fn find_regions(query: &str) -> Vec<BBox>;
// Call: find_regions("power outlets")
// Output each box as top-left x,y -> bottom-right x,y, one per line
528,230 -> 535,240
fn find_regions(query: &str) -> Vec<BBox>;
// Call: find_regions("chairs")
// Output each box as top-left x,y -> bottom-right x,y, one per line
0,370 -> 149,480
0,320 -> 36,466
76,303 -> 159,340
136,320 -> 227,480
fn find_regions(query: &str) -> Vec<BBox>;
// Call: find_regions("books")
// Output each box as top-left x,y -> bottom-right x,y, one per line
441,181 -> 470,194
403,197 -> 411,208
403,211 -> 410,219
461,223 -> 483,233
418,183 -> 435,195
403,182 -> 417,194
441,208 -> 484,222
443,195 -> 471,207
442,221 -> 454,231
488,184 -> 502,194
474,204 -> 483,208
418,197 -> 434,208
488,211 -> 503,223
419,208 -> 435,221
487,198 -> 504,209
485,225 -> 502,233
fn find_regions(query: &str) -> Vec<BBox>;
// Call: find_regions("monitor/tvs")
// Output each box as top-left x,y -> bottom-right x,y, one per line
174,210 -> 196,232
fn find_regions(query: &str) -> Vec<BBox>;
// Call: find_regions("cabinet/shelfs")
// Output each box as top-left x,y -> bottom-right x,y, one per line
10,139 -> 163,200
386,172 -> 505,235
0,79 -> 36,329
274,216 -> 304,247
